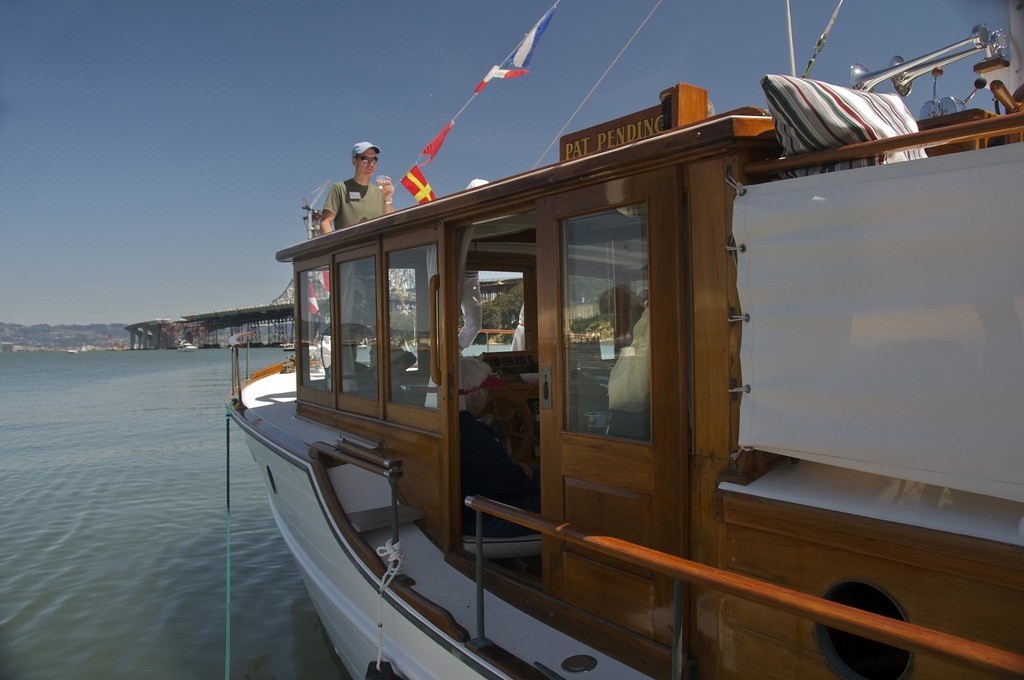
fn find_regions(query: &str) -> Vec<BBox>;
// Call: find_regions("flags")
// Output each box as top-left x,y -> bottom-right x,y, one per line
308,273 -> 320,317
401,165 -> 435,205
505,6 -> 557,67
317,271 -> 330,294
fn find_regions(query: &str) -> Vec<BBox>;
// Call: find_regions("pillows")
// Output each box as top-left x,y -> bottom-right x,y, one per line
760,74 -> 928,180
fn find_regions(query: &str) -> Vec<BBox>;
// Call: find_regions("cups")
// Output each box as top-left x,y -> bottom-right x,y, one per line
375,174 -> 386,189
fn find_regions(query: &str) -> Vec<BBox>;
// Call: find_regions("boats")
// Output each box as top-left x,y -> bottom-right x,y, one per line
282,343 -> 296,351
224,0 -> 1024,680
176,340 -> 198,353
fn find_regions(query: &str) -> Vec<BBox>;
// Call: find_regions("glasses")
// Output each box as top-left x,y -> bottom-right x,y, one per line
356,157 -> 378,163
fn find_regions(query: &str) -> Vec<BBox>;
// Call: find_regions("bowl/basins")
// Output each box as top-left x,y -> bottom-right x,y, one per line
519,373 -> 539,384
584,411 -> 608,428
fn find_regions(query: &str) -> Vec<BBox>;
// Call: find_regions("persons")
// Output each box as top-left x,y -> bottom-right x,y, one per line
324,343 -> 417,404
427,179 -> 490,351
438,357 -> 540,538
321,141 -> 395,391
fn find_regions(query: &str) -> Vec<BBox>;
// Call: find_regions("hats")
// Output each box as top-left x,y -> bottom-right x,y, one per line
363,344 -> 416,382
352,142 -> 380,157
